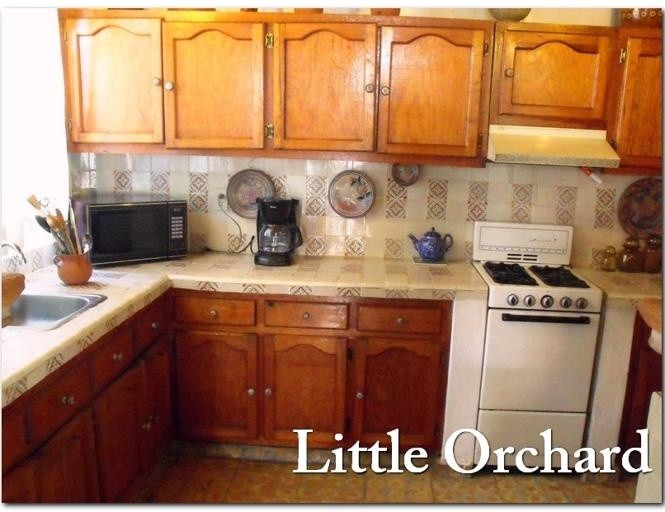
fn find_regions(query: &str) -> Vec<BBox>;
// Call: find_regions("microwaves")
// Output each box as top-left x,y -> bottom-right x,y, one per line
71,194 -> 188,266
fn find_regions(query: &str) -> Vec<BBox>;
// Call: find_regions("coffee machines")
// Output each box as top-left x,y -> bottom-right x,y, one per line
252,195 -> 303,265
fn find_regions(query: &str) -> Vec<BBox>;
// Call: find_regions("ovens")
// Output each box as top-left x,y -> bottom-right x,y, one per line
472,305 -> 603,468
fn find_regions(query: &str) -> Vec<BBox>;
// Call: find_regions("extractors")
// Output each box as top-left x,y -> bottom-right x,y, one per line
482,122 -> 624,168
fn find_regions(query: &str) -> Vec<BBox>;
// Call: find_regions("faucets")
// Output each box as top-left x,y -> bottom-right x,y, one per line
1,241 -> 28,265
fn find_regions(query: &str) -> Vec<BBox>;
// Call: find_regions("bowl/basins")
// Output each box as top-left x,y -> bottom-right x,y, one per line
487,9 -> 531,25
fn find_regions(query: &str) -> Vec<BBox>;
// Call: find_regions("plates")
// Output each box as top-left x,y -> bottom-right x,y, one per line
329,170 -> 376,218
391,162 -> 422,186
226,170 -> 274,220
615,178 -> 661,236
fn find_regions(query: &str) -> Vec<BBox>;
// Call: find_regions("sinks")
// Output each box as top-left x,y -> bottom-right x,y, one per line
5,289 -> 108,331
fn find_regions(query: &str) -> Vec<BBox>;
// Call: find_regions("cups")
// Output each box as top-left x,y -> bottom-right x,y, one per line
52,250 -> 92,286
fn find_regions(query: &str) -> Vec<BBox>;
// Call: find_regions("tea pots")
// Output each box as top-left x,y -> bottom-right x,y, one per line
408,227 -> 453,261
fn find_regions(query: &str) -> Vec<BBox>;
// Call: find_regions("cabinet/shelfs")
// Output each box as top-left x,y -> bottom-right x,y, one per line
268,12 -> 497,170
488,19 -> 615,133
170,288 -> 354,464
351,297 -> 454,470
55,6 -> 270,160
3,287 -> 173,502
601,27 -> 664,179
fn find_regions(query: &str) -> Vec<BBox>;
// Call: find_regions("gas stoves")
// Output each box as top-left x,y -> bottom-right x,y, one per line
473,261 -> 604,314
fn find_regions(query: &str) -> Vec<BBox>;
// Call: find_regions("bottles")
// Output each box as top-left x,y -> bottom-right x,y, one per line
603,237 -> 662,273
